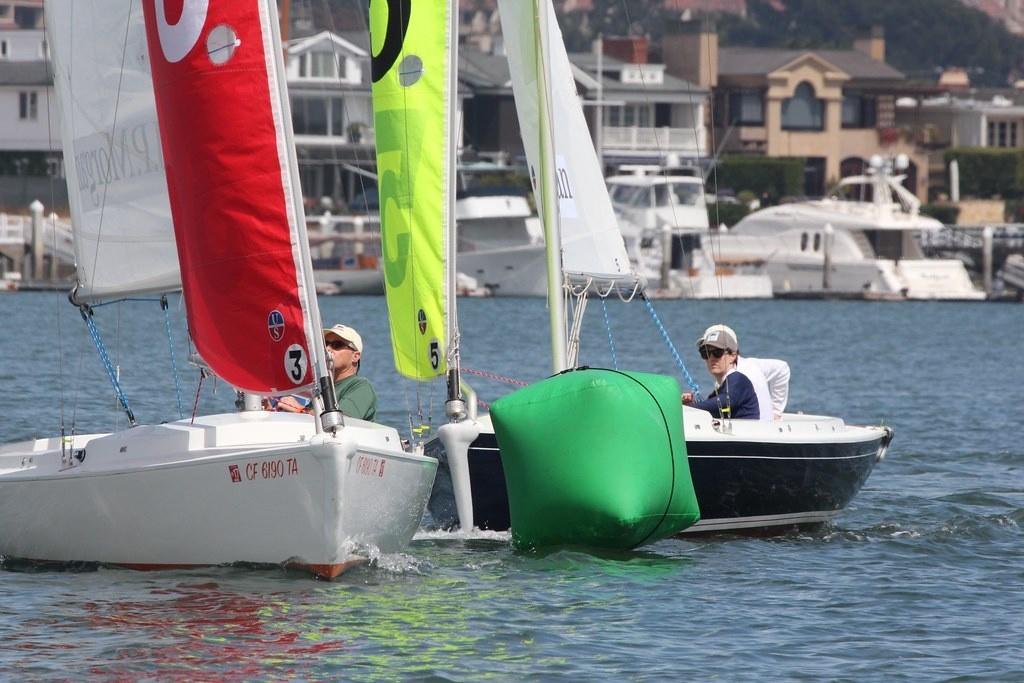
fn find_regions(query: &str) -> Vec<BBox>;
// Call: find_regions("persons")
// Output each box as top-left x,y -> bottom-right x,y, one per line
259,324 -> 378,423
681,330 -> 759,422
693,324 -> 792,422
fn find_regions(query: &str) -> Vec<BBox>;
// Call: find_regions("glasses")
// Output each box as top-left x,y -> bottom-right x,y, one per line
325,340 -> 357,355
700,348 -> 734,359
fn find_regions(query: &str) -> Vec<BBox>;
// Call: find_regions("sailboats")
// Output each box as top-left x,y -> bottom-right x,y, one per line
0,4 -> 437,580
362,0 -> 897,549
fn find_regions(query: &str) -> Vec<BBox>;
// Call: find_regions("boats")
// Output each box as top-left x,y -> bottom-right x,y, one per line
443,156 -> 982,305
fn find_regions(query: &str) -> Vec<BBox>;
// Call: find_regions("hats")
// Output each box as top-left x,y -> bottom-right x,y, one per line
696,324 -> 738,353
323,323 -> 363,354
